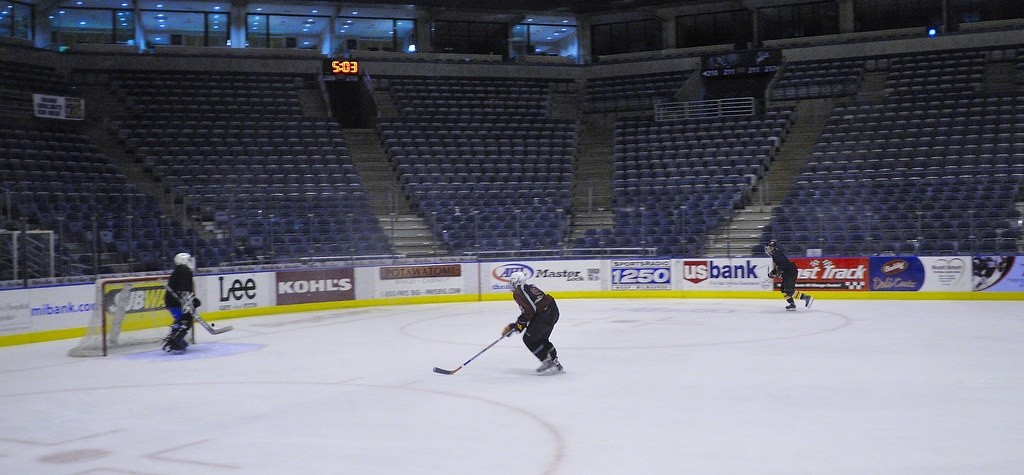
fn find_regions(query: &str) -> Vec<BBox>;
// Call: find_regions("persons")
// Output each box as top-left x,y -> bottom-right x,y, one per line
502,272 -> 563,372
765,240 -> 814,311
165,253 -> 200,355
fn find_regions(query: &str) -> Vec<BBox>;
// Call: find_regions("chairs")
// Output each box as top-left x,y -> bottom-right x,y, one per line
0,42 -> 1024,291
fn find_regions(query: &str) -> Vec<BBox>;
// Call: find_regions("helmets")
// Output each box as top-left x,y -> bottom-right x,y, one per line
174,253 -> 191,266
765,241 -> 778,257
509,272 -> 526,291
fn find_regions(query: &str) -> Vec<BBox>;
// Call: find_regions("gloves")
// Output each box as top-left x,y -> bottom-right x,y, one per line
181,292 -> 194,314
503,324 -> 514,337
190,298 -> 201,307
776,274 -> 781,278
515,320 -> 527,333
768,273 -> 774,278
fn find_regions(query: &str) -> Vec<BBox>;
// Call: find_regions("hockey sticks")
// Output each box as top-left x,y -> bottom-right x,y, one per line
432,329 -> 514,377
158,279 -> 234,335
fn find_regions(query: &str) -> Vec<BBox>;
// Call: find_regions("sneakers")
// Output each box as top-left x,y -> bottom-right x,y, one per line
536,359 -> 557,375
172,344 -> 184,354
550,361 -> 563,373
805,295 -> 814,308
785,304 -> 796,311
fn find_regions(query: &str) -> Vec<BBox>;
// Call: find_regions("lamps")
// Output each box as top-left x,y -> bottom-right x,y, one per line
224,40 -> 232,47
926,25 -> 939,37
408,32 -> 416,52
